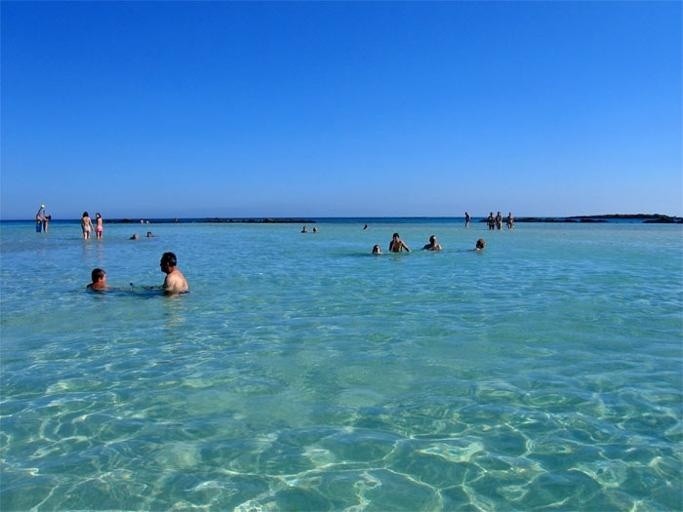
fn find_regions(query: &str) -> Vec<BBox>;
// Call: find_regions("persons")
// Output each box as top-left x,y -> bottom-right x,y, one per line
146,231 -> 153,237
95,213 -> 103,240
371,244 -> 382,255
473,238 -> 485,252
494,210 -> 502,231
85,268 -> 111,291
80,211 -> 94,240
485,211 -> 494,229
130,232 -> 138,240
423,234 -> 441,251
506,211 -> 512,230
300,225 -> 308,233
43,215 -> 51,233
159,252 -> 189,295
312,227 -> 318,232
36,204 -> 46,221
463,210 -> 470,228
388,234 -> 410,253
